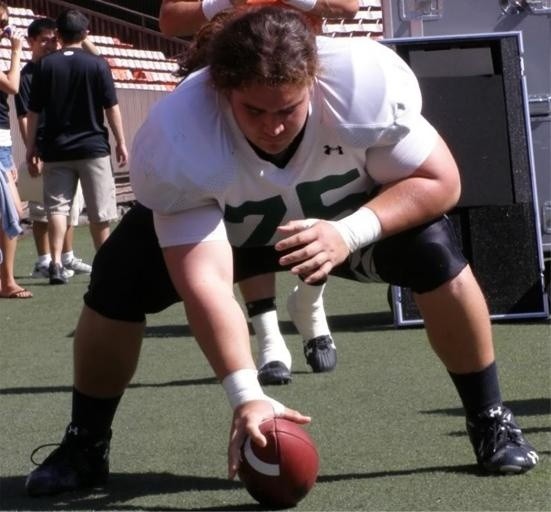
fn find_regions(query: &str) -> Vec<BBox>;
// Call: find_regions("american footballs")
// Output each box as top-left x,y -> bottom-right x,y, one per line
238,417 -> 319,508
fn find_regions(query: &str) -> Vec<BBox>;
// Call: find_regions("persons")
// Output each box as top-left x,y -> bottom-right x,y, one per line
15,15 -> 96,278
0,0 -> 38,299
156,0 -> 359,387
21,9 -> 130,285
24,10 -> 541,508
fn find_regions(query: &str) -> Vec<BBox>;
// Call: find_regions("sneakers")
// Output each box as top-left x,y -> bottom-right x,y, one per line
254,345 -> 293,387
32,261 -> 75,279
22,421 -> 114,508
48,260 -> 67,285
464,405 -> 540,476
284,283 -> 337,372
62,257 -> 93,273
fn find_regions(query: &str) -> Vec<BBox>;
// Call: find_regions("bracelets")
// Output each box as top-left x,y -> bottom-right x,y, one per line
286,1 -> 319,14
202,1 -> 235,23
12,46 -> 21,52
221,368 -> 264,411
337,207 -> 384,254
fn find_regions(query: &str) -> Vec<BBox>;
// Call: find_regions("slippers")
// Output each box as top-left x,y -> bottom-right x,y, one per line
0,286 -> 33,299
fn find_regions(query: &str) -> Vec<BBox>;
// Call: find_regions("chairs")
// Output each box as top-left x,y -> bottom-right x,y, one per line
0,0 -> 187,92
313,0 -> 384,43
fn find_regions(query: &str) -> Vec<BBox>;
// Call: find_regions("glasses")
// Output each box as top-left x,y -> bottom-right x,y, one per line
0,20 -> 9,28
